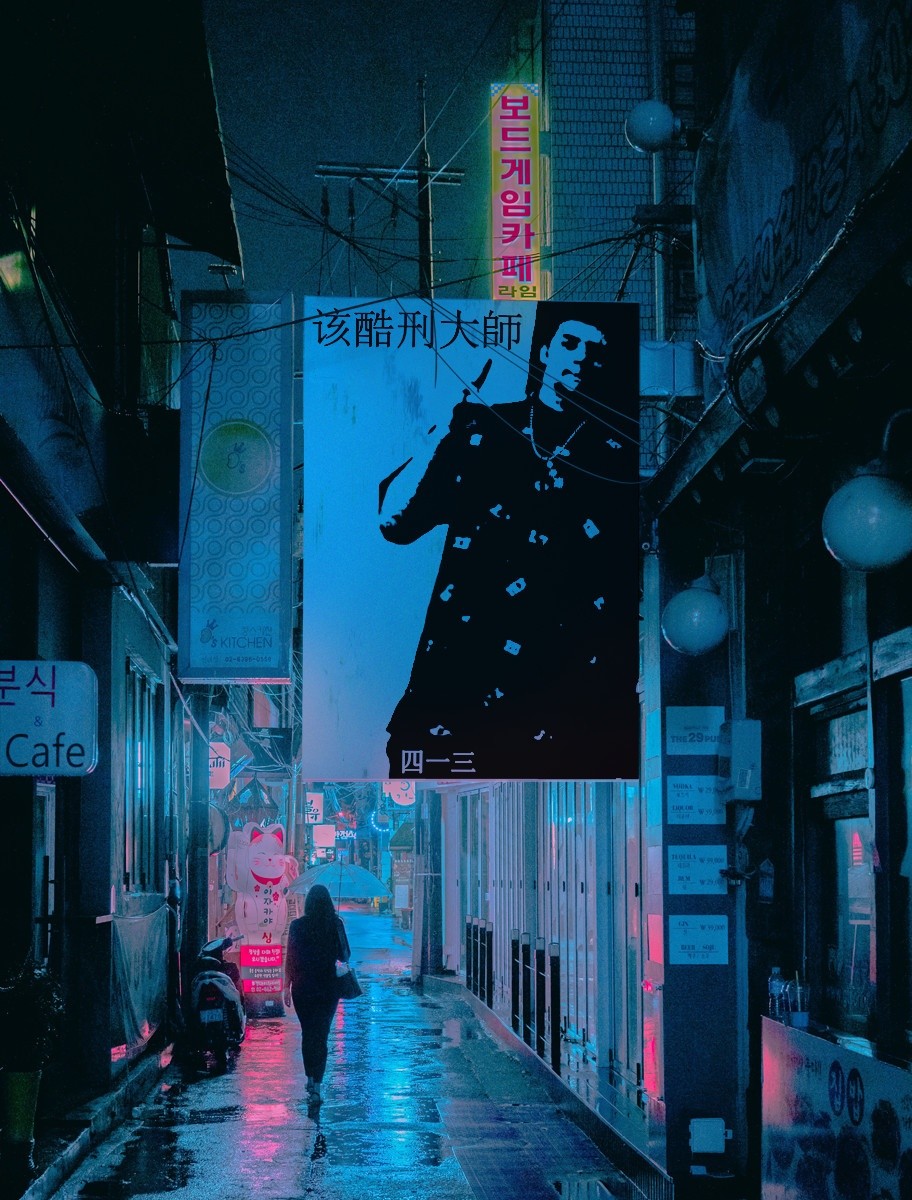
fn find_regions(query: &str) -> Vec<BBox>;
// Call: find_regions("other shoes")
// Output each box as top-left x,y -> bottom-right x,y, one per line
304,1083 -> 314,1092
306,1092 -> 324,1106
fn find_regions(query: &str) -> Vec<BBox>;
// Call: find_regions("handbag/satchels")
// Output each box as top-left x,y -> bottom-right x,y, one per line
337,965 -> 363,999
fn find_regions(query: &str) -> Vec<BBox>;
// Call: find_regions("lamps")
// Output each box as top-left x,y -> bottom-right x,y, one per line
823,408 -> 912,571
625,100 -> 713,153
661,544 -> 728,654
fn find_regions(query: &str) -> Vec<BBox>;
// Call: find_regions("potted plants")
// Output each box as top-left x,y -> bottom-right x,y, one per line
0,962 -> 71,1173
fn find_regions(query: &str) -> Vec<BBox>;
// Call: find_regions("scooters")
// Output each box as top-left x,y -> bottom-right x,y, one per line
186,931 -> 249,1069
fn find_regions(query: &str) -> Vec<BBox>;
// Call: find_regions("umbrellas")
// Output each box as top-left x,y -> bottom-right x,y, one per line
286,852 -> 394,919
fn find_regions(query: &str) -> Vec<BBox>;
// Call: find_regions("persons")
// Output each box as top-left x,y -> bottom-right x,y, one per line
282,877 -> 352,1108
